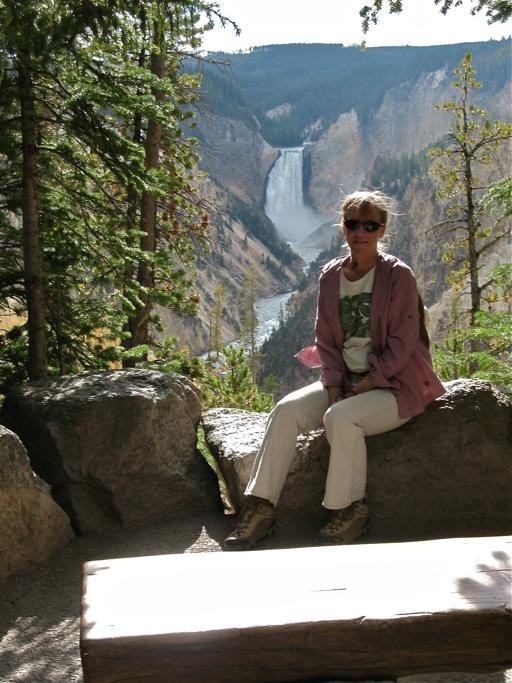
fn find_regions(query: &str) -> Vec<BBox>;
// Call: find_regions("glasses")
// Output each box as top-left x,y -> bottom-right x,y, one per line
342,219 -> 385,233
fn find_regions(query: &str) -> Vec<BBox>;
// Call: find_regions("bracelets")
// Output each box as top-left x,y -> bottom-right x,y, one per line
328,394 -> 343,406
346,389 -> 356,396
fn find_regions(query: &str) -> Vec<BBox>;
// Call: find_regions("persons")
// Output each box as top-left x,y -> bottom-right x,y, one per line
223,188 -> 446,550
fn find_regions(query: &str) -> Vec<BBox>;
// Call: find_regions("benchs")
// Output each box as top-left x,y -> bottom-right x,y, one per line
80,535 -> 512,683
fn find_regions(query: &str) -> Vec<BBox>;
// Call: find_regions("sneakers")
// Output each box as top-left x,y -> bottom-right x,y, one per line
318,494 -> 370,544
223,496 -> 279,551
